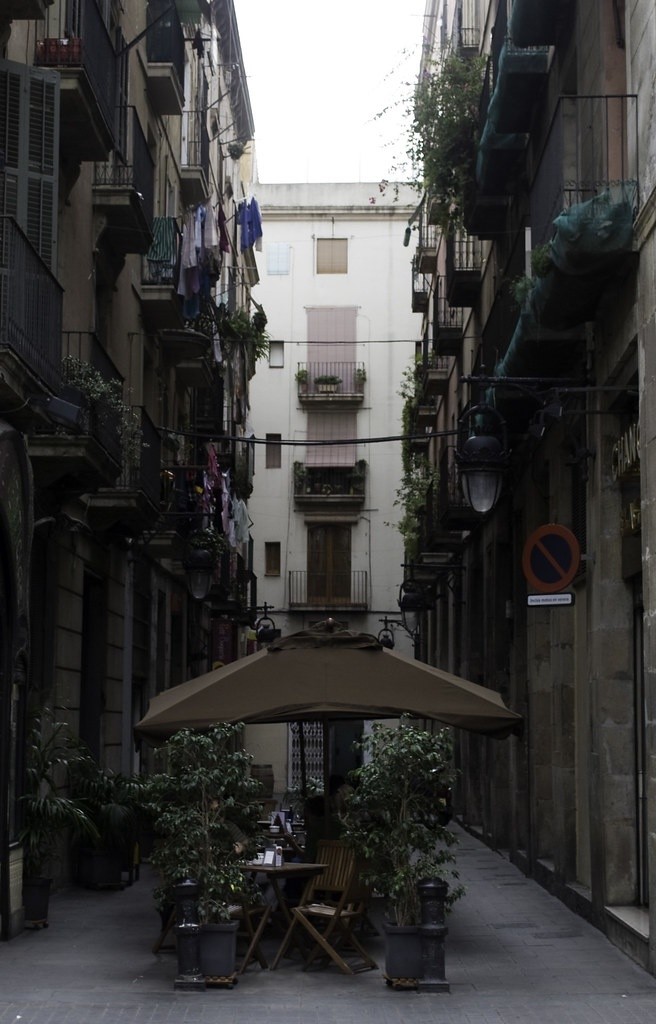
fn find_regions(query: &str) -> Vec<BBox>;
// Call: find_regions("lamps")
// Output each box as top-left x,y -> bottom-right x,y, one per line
398,561 -> 479,644
378,616 -> 402,651
456,374 -> 587,519
45,398 -> 83,434
240,604 -> 281,649
164,510 -> 223,604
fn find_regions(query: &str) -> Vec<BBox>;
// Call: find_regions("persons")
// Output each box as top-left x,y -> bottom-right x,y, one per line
325,775 -> 350,815
208,801 -> 249,862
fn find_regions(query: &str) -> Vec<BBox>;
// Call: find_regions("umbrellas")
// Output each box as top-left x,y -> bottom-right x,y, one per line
135,616 -> 519,797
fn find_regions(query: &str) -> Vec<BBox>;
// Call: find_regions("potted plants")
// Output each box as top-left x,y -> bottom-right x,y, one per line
295,370 -> 308,392
352,368 -> 366,394
315,374 -> 343,393
334,711 -> 465,979
18,687 -> 262,978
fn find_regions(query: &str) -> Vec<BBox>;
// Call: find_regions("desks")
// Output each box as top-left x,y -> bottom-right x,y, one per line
257,821 -> 307,831
228,860 -> 329,975
283,846 -> 305,861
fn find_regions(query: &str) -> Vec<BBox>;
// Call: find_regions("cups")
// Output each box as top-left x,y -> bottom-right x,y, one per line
270,826 -> 279,832
257,853 -> 264,864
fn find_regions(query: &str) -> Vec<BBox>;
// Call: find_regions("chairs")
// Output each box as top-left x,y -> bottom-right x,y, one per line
154,839 -> 379,979
